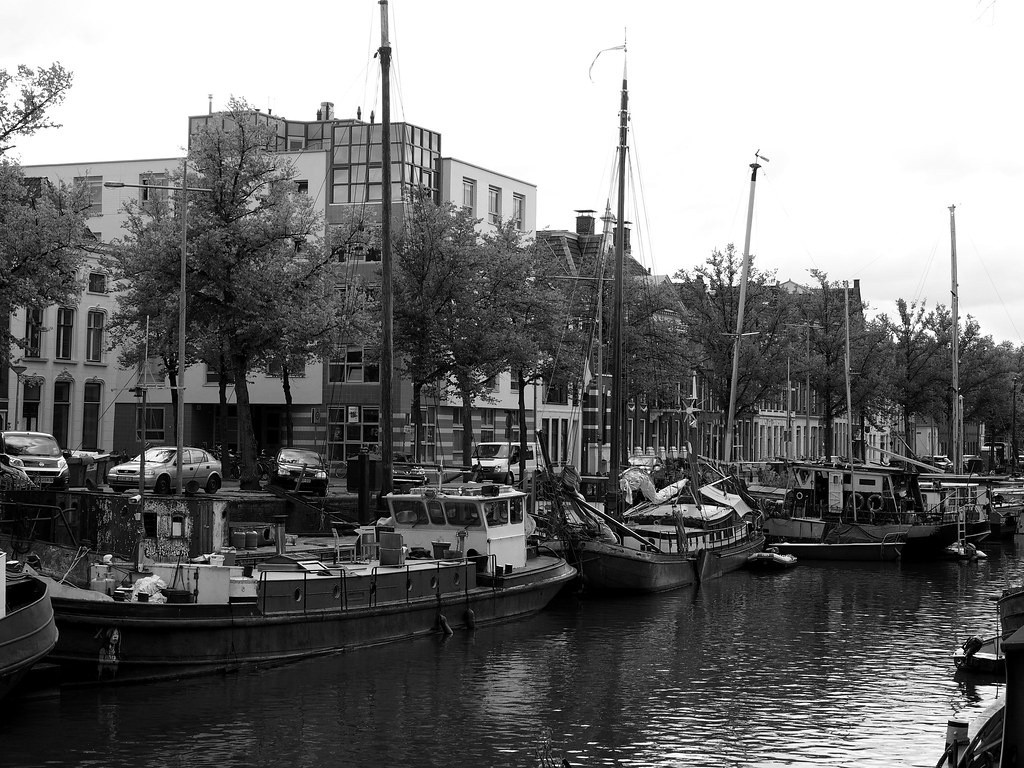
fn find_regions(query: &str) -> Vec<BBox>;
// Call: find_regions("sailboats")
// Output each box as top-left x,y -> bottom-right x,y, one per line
517,23 -> 764,593
22,1 -> 576,684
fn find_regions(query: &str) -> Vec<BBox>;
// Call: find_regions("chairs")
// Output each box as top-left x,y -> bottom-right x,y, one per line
380,532 -> 410,568
359,526 -> 379,562
331,528 -> 356,565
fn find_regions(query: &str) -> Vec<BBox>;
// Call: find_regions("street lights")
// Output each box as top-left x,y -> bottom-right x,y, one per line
105,158 -> 215,493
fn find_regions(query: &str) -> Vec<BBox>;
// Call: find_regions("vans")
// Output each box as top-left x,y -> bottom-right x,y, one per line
471,440 -> 544,486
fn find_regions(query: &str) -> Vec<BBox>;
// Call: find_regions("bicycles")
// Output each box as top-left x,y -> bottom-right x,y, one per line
201,443 -> 271,482
335,452 -> 359,478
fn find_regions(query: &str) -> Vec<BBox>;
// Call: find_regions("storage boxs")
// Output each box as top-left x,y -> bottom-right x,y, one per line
221,546 -> 236,566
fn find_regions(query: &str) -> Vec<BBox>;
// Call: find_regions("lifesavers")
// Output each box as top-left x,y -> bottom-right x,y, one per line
848,493 -> 864,510
867,494 -> 884,512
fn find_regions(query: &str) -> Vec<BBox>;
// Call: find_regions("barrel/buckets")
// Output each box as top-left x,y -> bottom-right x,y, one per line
430,541 -> 451,560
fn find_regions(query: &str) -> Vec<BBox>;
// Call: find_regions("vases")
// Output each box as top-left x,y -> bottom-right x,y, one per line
444,549 -> 454,559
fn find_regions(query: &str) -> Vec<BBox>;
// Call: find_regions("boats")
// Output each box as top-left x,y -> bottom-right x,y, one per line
746,547 -> 798,573
0,537 -> 61,688
914,201 -> 1023,534
743,278 -> 992,565
715,148 -> 828,546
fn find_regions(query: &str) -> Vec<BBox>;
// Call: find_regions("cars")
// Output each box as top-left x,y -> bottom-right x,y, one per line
962,455 -> 979,465
627,454 -> 667,484
106,446 -> 222,493
379,451 -> 429,492
0,429 -> 71,488
921,455 -> 953,471
266,446 -> 329,497
821,455 -> 848,463
